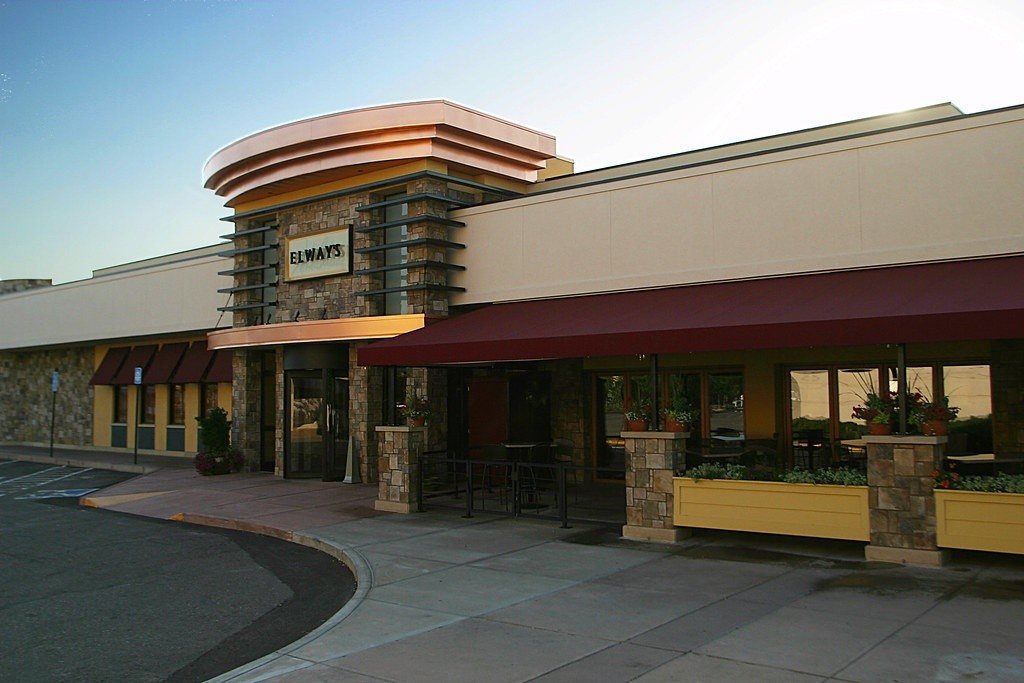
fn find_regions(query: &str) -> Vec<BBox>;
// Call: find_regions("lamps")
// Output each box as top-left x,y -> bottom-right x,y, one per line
266,313 -> 272,324
318,307 -> 326,320
252,315 -> 259,327
293,310 -> 300,322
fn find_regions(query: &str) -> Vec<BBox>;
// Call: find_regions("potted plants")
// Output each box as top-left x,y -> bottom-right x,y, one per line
196,406 -> 232,475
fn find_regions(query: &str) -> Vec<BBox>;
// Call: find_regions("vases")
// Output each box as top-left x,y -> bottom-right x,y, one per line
923,419 -> 948,435
629,417 -> 650,430
868,417 -> 893,435
407,412 -> 424,428
665,419 -> 690,433
229,462 -> 242,472
200,467 -> 213,475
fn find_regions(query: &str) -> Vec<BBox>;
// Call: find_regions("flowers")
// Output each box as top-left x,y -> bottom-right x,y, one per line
904,395 -> 955,425
227,450 -> 245,468
855,391 -> 899,421
400,386 -> 432,416
664,397 -> 701,424
194,453 -> 216,471
625,400 -> 655,422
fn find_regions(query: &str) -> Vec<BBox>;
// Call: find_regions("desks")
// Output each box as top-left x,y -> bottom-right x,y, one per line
684,447 -> 746,470
945,451 -> 1024,476
498,440 -> 571,507
